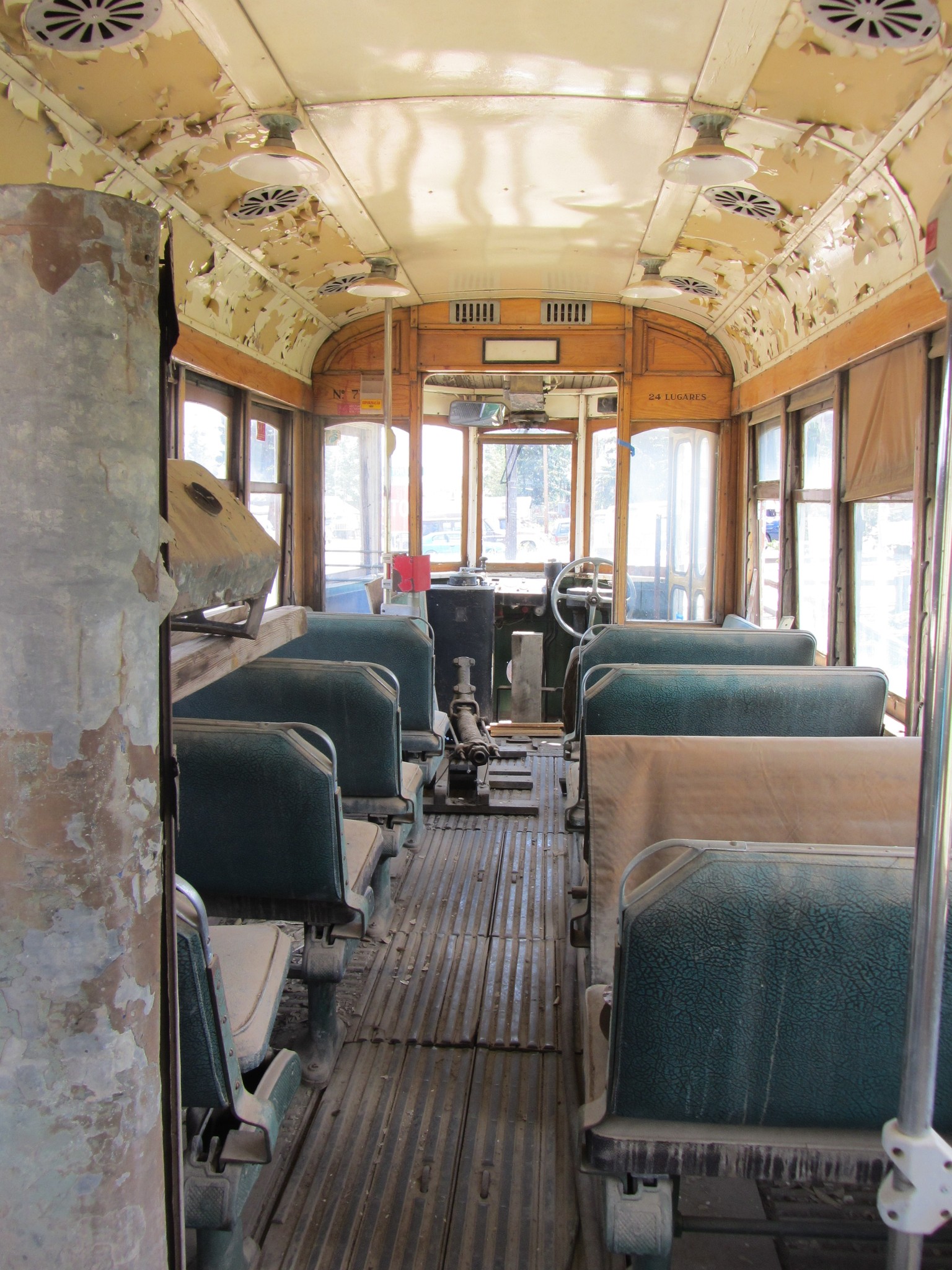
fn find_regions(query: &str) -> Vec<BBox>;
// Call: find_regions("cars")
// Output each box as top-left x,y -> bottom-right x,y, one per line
415,514 -> 570,562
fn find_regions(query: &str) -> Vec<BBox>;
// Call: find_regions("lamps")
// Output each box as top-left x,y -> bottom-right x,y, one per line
619,259 -> 681,299
659,114 -> 757,187
229,114 -> 330,186
346,257 -> 411,297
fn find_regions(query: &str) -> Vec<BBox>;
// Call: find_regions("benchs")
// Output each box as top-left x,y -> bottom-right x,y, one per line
563,624 -> 952,1270
171,615 -> 449,1270
